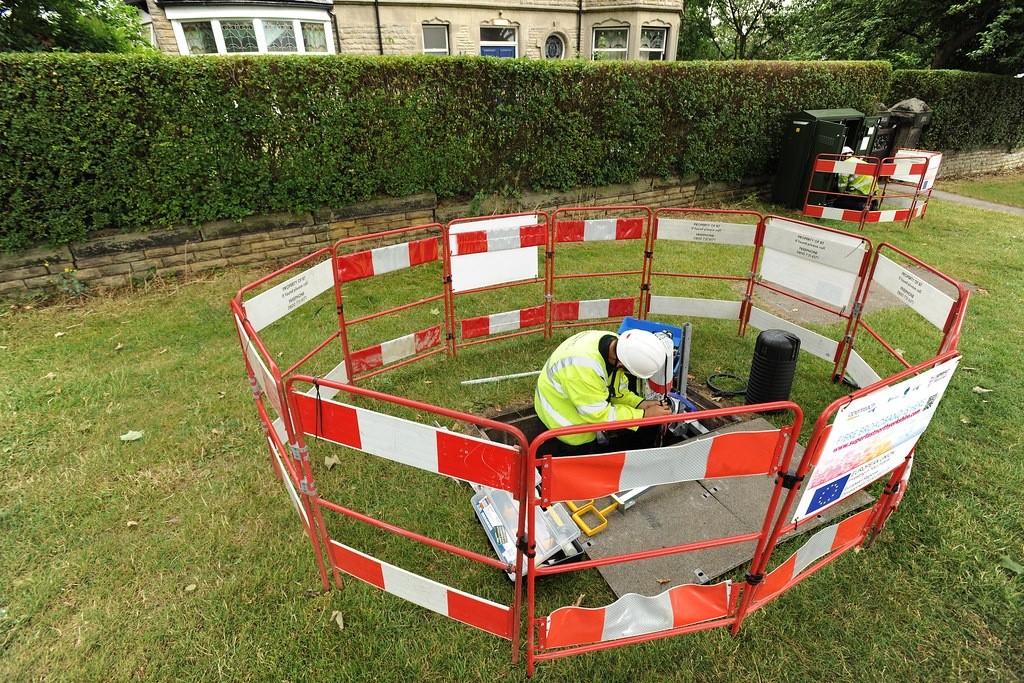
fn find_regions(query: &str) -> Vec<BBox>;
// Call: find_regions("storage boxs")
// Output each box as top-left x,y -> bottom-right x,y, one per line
470,466 -> 585,588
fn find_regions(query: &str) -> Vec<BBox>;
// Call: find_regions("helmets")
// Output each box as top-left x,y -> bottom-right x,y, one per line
616,328 -> 666,379
840,146 -> 854,160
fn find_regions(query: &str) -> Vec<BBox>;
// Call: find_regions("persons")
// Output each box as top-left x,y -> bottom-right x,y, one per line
831,146 -> 879,210
534,329 -> 672,457
